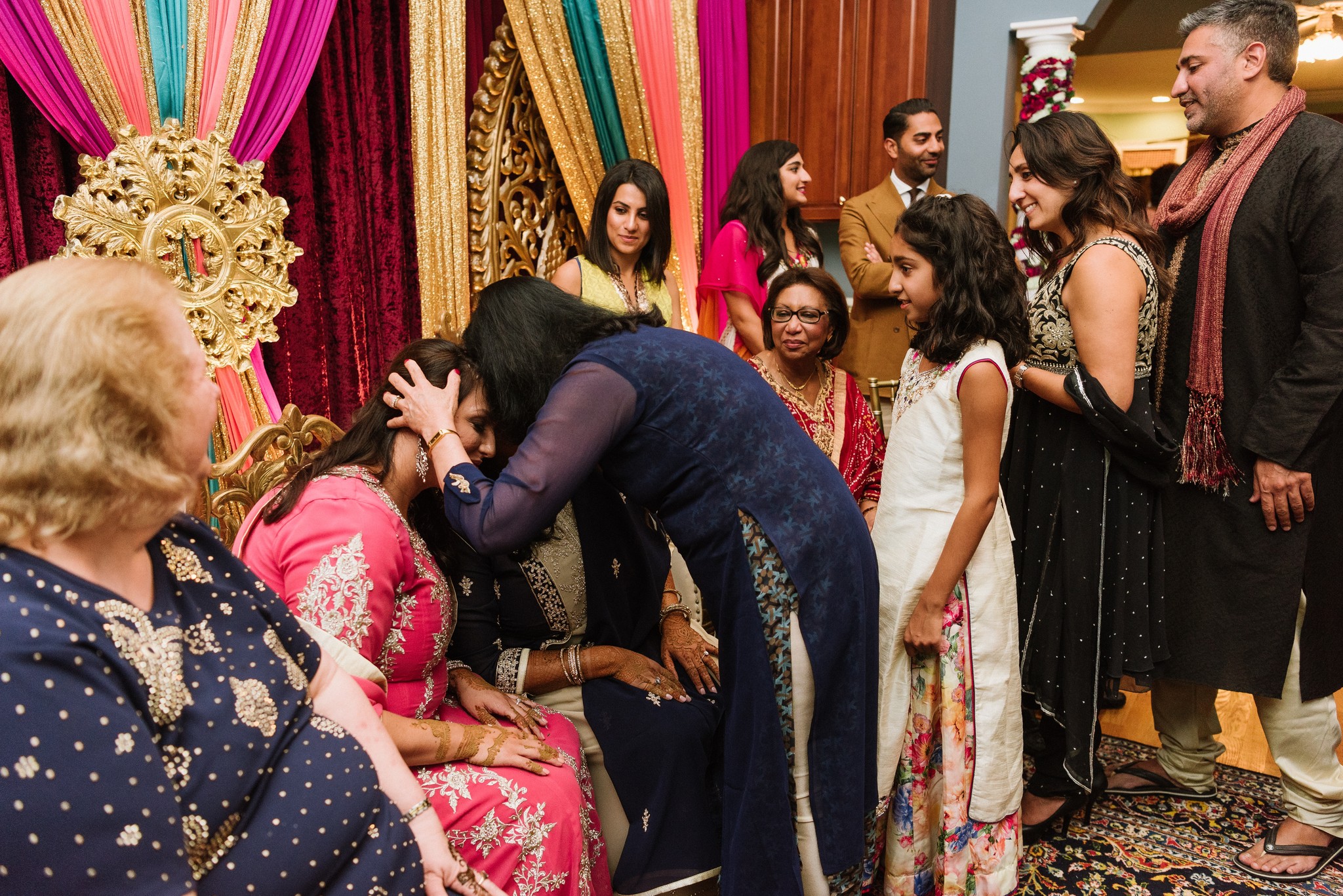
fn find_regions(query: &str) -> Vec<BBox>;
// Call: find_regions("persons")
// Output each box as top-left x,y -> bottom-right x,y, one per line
229,340 -> 618,896
741,267 -> 887,535
691,139 -> 826,363
1106,0 -> 1343,882
992,109 -> 1180,847
549,159 -> 672,327
867,191 -> 1022,896
1149,163 -> 1187,208
409,399 -> 724,896
833,97 -> 962,442
1,257 -> 522,896
384,279 -> 881,896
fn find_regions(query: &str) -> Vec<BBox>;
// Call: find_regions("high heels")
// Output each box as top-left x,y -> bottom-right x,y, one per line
1022,759 -> 1108,839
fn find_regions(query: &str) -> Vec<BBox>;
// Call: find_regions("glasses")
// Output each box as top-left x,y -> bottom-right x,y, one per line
770,307 -> 832,324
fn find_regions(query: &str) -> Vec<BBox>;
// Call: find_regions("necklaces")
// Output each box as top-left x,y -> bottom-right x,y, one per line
603,267 -> 648,315
773,354 -> 833,426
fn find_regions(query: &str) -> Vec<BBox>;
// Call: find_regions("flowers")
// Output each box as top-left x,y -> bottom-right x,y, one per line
1012,56 -> 1067,277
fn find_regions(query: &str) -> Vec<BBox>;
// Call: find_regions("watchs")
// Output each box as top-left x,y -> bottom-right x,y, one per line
425,428 -> 461,460
1013,363 -> 1034,389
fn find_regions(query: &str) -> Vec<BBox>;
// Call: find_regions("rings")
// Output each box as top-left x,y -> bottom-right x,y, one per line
516,698 -> 521,705
701,651 -> 709,661
477,869 -> 489,885
654,676 -> 661,686
392,395 -> 402,410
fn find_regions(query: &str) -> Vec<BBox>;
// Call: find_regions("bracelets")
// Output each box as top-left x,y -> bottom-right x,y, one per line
401,796 -> 432,825
662,588 -> 682,603
658,602 -> 692,634
560,644 -> 586,685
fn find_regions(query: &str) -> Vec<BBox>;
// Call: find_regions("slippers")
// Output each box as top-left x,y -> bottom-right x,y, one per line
1233,815 -> 1343,882
1105,757 -> 1218,800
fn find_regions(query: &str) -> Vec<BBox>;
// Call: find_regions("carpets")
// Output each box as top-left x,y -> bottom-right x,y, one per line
1015,732 -> 1343,896
